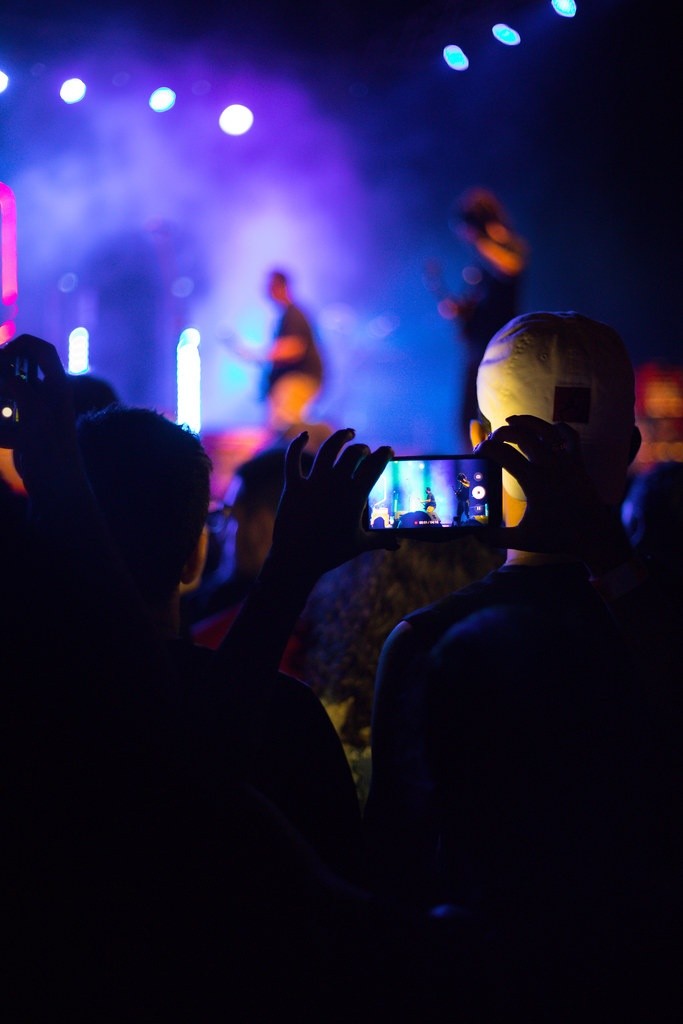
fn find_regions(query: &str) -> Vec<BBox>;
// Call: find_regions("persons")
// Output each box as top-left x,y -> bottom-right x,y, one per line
452,472 -> 471,522
421,487 -> 437,512
0,190 -> 682,1024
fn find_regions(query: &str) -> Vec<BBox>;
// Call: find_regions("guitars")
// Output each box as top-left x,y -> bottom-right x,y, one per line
416,497 -> 425,509
452,485 -> 461,498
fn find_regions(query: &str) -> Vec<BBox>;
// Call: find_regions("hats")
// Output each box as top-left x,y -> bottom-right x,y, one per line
476,310 -> 636,507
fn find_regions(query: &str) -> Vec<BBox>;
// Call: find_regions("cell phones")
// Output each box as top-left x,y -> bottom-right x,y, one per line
362,454 -> 503,534
